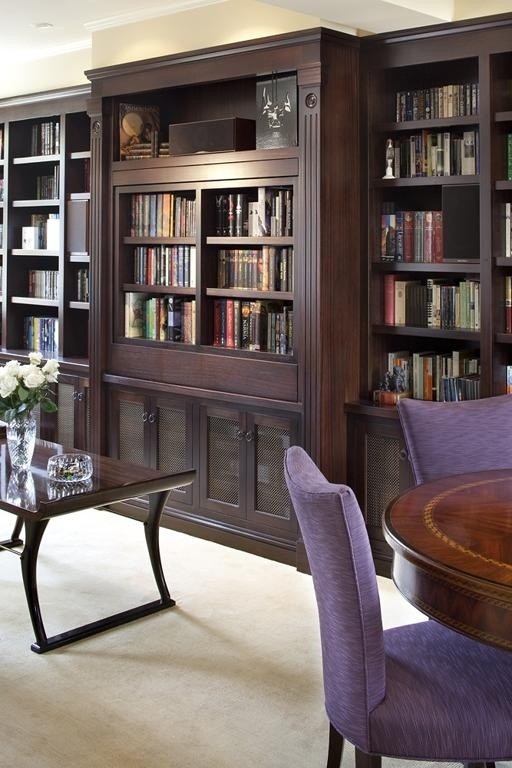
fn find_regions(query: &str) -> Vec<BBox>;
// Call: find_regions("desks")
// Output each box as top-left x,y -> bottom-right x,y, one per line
380,468 -> 512,652
0,436 -> 196,654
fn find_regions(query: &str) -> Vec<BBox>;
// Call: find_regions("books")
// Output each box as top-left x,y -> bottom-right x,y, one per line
133,245 -> 196,288
130,194 -> 193,238
506,364 -> 512,395
371,80 -> 481,407
18,121 -> 89,354
507,133 -> 512,181
215,187 -> 293,237
120,102 -> 168,160
505,276 -> 512,335
218,245 -> 294,292
213,299 -> 295,356
506,202 -> 512,258
124,293 -> 196,344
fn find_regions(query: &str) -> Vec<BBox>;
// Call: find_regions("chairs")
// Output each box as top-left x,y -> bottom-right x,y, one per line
283,445 -> 512,768
